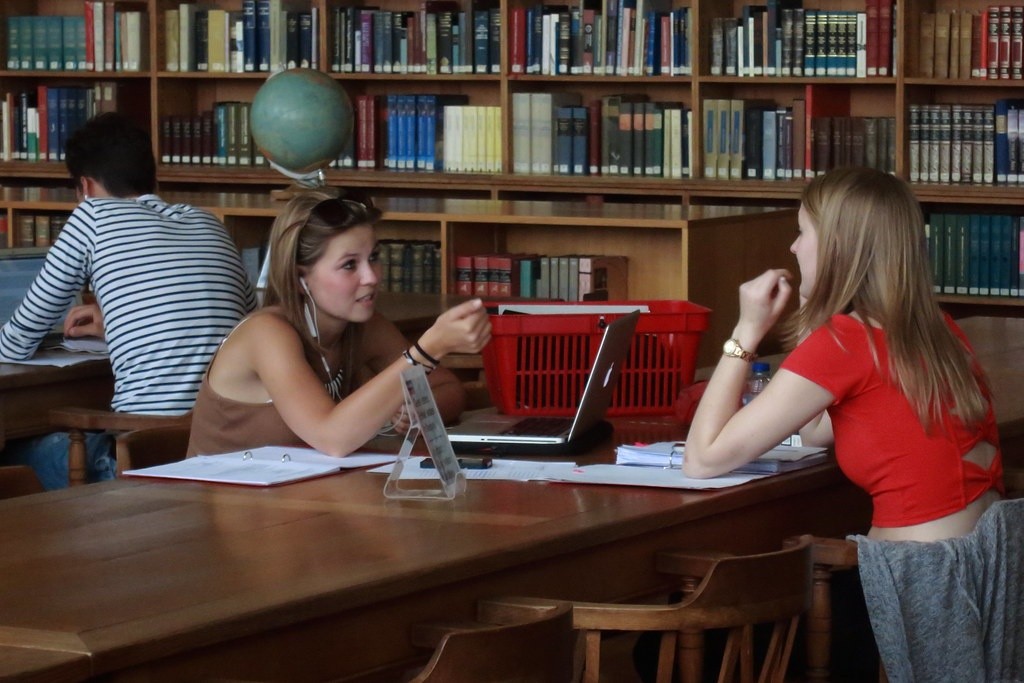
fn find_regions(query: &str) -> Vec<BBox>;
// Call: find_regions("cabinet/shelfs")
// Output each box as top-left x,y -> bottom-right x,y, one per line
0,0 -> 1024,366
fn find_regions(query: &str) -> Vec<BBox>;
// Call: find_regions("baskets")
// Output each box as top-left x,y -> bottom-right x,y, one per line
482,298 -> 711,417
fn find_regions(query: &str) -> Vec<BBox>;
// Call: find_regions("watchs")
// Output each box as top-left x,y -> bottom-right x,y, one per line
722,336 -> 759,367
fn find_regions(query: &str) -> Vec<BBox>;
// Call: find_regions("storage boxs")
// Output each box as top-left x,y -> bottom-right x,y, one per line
459,299 -> 714,418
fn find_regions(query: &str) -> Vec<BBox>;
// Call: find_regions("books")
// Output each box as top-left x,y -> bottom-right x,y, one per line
548,442 -> 827,510
0,0 -> 1024,307
497,303 -> 650,406
122,447 -> 398,486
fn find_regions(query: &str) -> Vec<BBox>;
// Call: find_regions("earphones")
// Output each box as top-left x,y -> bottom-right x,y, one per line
299,276 -> 311,294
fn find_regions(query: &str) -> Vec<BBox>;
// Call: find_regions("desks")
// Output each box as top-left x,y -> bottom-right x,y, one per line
0,291 -> 135,447
0,400 -> 875,683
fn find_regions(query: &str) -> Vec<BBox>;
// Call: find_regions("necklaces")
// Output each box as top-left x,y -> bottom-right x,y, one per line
317,368 -> 347,399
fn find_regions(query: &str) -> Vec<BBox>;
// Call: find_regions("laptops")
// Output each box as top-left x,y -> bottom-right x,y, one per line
446,308 -> 641,445
0,246 -> 87,348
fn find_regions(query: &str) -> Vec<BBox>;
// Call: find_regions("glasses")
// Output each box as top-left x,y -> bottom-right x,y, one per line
301,194 -> 372,226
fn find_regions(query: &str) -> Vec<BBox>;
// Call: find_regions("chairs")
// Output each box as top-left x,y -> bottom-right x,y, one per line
0,391 -> 1024,683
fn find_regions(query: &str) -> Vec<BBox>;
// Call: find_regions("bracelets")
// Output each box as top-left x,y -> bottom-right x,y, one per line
401,342 -> 439,375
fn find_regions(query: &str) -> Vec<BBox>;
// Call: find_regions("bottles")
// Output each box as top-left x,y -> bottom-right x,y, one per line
742,362 -> 770,411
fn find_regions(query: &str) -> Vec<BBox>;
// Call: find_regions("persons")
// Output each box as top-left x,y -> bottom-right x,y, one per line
0,113 -> 256,494
632,166 -> 1002,682
186,191 -> 492,460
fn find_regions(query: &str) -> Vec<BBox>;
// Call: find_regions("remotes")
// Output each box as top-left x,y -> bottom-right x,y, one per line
421,457 -> 493,469
